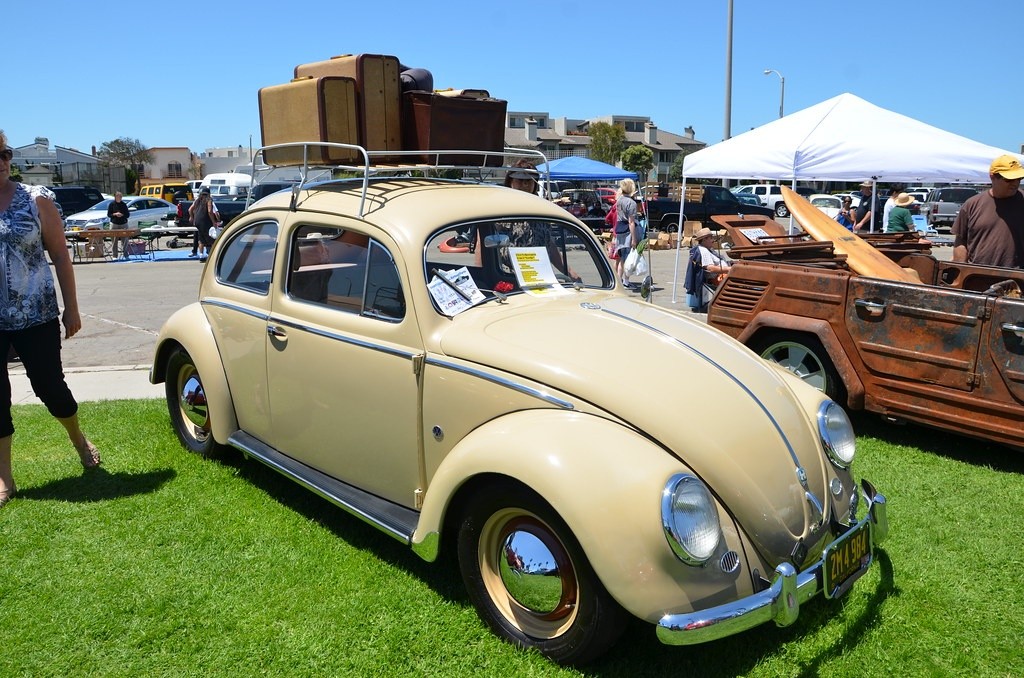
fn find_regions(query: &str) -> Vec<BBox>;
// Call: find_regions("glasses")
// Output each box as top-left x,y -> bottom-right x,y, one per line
994,174 -> 1024,182
0,149 -> 12,161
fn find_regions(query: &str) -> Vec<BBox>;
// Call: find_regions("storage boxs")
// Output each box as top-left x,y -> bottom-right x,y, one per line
85,229 -> 104,258
600,221 -> 730,251
402,101 -> 508,166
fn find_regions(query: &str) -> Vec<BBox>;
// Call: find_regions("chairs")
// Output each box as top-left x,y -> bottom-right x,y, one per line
137,220 -> 162,250
910,215 -> 939,236
328,257 -> 399,314
689,261 -> 720,312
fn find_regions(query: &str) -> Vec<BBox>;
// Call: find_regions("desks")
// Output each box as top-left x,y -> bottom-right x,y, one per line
141,226 -> 197,260
64,228 -> 139,263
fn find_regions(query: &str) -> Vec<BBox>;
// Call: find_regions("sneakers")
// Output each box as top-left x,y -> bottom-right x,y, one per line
623,282 -> 637,289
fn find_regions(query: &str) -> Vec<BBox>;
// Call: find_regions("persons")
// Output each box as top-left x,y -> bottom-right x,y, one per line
588,202 -> 606,233
474,160 -> 581,281
107,192 -> 129,258
188,188 -> 220,259
882,183 -> 915,232
0,131 -> 100,506
695,228 -> 739,284
950,155 -> 1024,270
837,182 -> 880,233
606,178 -> 637,289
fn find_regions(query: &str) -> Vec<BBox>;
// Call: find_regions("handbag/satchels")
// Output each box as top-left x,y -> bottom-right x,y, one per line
624,247 -> 641,277
636,256 -> 647,276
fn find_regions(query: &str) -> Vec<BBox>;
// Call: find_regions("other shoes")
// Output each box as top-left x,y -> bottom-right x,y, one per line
200,254 -> 204,258
189,253 -> 197,257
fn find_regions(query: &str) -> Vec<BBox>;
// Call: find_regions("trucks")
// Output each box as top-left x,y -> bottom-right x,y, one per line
705,212 -> 1024,451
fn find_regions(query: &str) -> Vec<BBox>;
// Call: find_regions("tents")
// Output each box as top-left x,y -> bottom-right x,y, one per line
673,92 -> 1024,301
536,156 -> 648,224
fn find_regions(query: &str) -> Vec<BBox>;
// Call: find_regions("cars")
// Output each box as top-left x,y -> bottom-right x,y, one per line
533,179 -> 979,243
43,163 -> 332,241
147,142 -> 891,666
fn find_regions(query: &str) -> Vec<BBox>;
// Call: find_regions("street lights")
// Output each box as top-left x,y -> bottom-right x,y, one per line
764,69 -> 785,118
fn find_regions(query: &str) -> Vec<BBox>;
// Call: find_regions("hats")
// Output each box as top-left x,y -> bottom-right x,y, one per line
695,227 -> 713,241
859,181 -> 873,187
509,171 -> 538,180
201,188 -> 210,195
895,192 -> 915,206
989,155 -> 1024,180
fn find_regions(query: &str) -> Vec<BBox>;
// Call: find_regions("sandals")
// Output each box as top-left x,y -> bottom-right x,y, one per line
74,434 -> 100,468
0,479 -> 17,508
